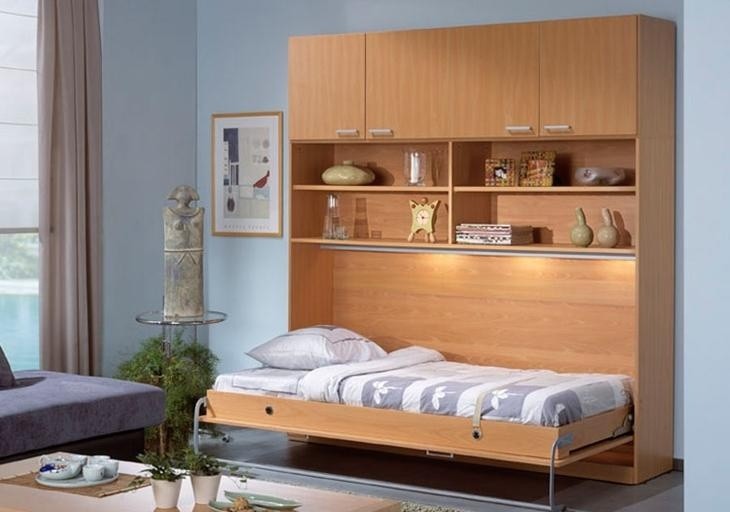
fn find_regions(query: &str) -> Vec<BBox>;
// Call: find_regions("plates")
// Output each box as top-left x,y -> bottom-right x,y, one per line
207,490 -> 303,512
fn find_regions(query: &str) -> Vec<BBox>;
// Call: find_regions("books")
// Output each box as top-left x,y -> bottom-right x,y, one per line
455,220 -> 536,248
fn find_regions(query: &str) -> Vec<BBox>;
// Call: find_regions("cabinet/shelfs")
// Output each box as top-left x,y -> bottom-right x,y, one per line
287,11 -> 636,141
292,140 -> 637,256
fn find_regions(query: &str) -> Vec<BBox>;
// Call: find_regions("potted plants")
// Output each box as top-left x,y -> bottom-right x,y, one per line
128,443 -> 262,512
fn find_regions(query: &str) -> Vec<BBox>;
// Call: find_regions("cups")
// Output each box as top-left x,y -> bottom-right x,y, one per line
84,456 -> 119,481
333,224 -> 348,239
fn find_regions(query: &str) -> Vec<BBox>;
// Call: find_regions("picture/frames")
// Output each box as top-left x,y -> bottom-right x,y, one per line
208,113 -> 280,237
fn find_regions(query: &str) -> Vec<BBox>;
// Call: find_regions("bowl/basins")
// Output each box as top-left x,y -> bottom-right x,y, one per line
38,452 -> 88,479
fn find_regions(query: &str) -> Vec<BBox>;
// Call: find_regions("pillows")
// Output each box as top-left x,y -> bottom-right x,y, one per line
246,323 -> 393,368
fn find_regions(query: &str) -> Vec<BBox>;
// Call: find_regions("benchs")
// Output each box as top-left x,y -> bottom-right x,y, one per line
203,319 -> 633,512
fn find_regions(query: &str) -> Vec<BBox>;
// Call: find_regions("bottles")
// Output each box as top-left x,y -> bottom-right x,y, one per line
404,148 -> 426,186
571,206 -> 619,247
320,194 -> 342,238
